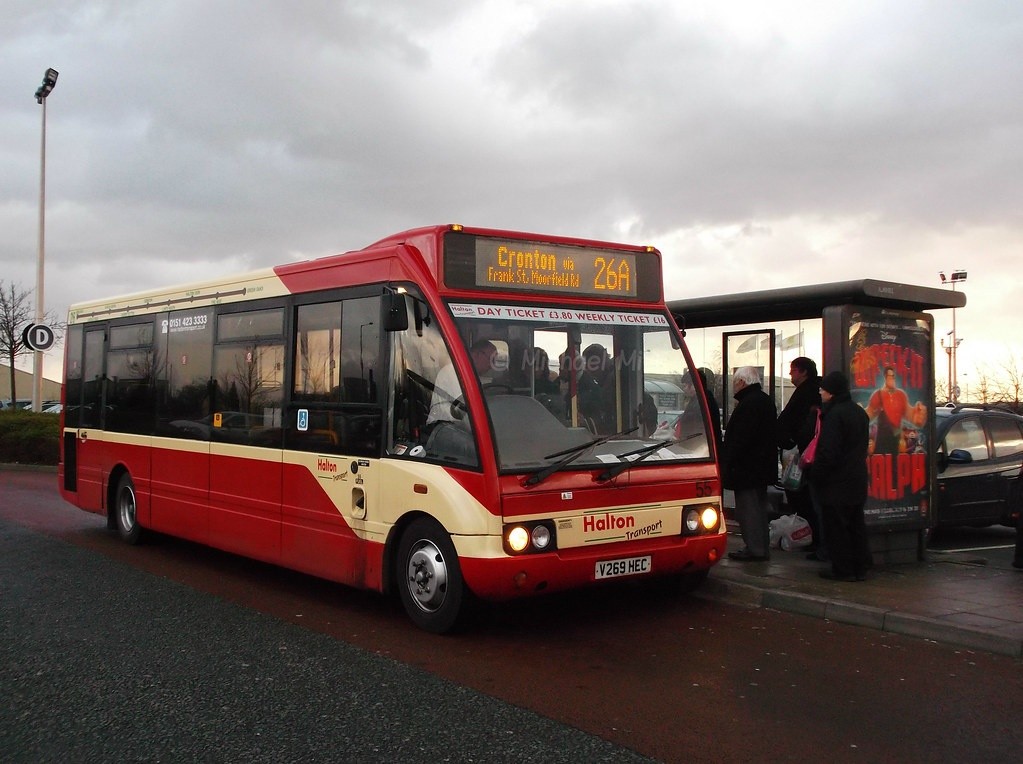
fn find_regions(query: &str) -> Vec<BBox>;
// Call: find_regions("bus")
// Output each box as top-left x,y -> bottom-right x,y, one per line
58,223 -> 729,631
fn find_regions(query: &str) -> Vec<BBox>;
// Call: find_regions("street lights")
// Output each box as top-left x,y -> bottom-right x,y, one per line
34,68 -> 60,414
941,330 -> 963,402
940,272 -> 968,402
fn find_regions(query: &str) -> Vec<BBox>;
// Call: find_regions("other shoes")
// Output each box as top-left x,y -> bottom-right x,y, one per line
845,569 -> 873,582
728,549 -> 756,561
807,545 -> 845,560
1013,555 -> 1023,569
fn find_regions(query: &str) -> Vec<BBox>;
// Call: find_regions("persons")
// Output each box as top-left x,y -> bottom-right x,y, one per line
424,338 -> 498,440
524,343 -> 659,439
675,357 -> 870,580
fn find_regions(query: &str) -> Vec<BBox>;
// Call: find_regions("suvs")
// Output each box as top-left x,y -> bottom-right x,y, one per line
933,403 -> 1023,526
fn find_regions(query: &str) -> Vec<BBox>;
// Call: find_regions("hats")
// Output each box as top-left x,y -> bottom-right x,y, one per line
820,370 -> 849,396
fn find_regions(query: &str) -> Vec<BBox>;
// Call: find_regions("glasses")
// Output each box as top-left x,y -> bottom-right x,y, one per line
479,350 -> 496,365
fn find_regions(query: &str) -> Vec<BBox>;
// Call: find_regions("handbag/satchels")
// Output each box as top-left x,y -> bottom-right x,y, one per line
798,408 -> 821,469
769,513 -> 814,552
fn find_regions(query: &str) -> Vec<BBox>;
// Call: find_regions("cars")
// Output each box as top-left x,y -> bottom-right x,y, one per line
935,402 -> 993,427
0,399 -> 63,413
280,401 -> 382,453
67,403 -> 119,414
168,411 -> 272,441
